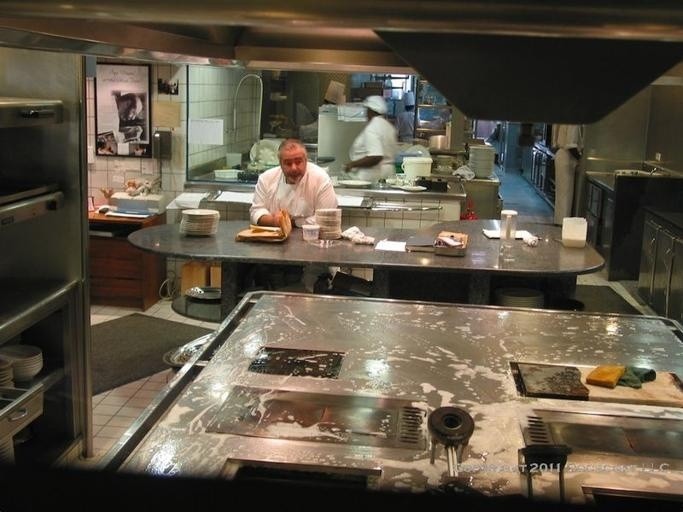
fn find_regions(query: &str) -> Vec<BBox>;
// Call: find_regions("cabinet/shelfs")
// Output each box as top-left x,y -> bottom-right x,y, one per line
1,46 -> 92,466
86,210 -> 166,311
578,169 -> 682,326
531,143 -> 558,208
413,79 -> 478,147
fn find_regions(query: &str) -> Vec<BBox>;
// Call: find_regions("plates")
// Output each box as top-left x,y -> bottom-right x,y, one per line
389,185 -> 427,192
3,343 -> 42,384
186,287 -> 222,303
469,146 -> 495,179
341,177 -> 375,191
0,355 -> 15,390
316,207 -> 342,239
437,230 -> 468,259
482,228 -> 534,239
179,209 -> 220,238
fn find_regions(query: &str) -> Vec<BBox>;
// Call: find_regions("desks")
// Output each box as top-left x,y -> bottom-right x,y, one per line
124,219 -> 605,323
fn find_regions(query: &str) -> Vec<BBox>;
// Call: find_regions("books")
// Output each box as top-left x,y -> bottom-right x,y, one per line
406,230 -> 469,257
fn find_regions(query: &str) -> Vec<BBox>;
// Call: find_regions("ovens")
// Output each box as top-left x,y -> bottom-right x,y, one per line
583,181 -> 602,250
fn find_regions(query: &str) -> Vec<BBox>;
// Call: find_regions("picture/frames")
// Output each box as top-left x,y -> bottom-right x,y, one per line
92,63 -> 152,158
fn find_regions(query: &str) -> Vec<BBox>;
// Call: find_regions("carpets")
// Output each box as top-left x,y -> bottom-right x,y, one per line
575,286 -> 643,315
90,313 -> 217,397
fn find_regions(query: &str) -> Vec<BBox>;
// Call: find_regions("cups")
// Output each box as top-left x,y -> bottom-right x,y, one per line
499,209 -> 518,249
302,225 -> 319,243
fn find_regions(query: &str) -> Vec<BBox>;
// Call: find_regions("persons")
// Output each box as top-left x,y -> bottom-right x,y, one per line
485,122 -> 505,165
395,97 -> 421,143
250,138 -> 337,227
343,95 -> 398,183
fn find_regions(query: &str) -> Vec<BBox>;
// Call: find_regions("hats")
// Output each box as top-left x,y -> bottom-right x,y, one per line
361,95 -> 389,115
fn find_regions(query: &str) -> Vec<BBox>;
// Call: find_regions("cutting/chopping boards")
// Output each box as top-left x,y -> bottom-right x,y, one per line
511,361 -> 683,409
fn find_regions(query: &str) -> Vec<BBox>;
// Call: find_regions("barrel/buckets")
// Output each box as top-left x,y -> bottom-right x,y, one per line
469,144 -> 496,178
400,157 -> 434,181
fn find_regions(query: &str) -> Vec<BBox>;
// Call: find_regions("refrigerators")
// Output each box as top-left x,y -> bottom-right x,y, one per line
318,107 -> 371,170
